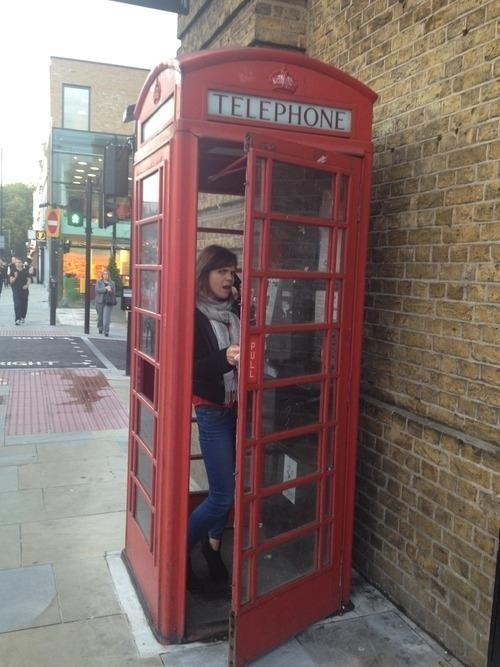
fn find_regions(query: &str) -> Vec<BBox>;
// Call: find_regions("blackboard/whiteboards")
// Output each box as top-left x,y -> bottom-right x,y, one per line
234,273 -> 241,308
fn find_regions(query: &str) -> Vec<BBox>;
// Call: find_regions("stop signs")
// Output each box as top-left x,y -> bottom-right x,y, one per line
45,209 -> 60,234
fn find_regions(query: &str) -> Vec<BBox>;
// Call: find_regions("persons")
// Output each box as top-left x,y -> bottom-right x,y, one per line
0,256 -> 35,296
9,256 -> 32,325
94,269 -> 116,337
188,243 -> 270,595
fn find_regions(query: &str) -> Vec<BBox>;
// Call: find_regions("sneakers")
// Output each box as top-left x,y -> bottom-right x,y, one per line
186,556 -> 204,594
99,328 -> 109,336
202,538 -> 228,581
15,318 -> 24,325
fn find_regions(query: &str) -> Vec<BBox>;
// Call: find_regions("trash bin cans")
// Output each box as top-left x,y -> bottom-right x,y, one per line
62,278 -> 79,301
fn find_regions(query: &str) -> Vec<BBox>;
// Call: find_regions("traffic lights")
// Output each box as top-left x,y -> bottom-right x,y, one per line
68,196 -> 84,227
99,192 -> 117,230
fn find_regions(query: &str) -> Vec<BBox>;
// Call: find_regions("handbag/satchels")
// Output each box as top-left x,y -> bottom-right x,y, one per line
105,292 -> 117,305
